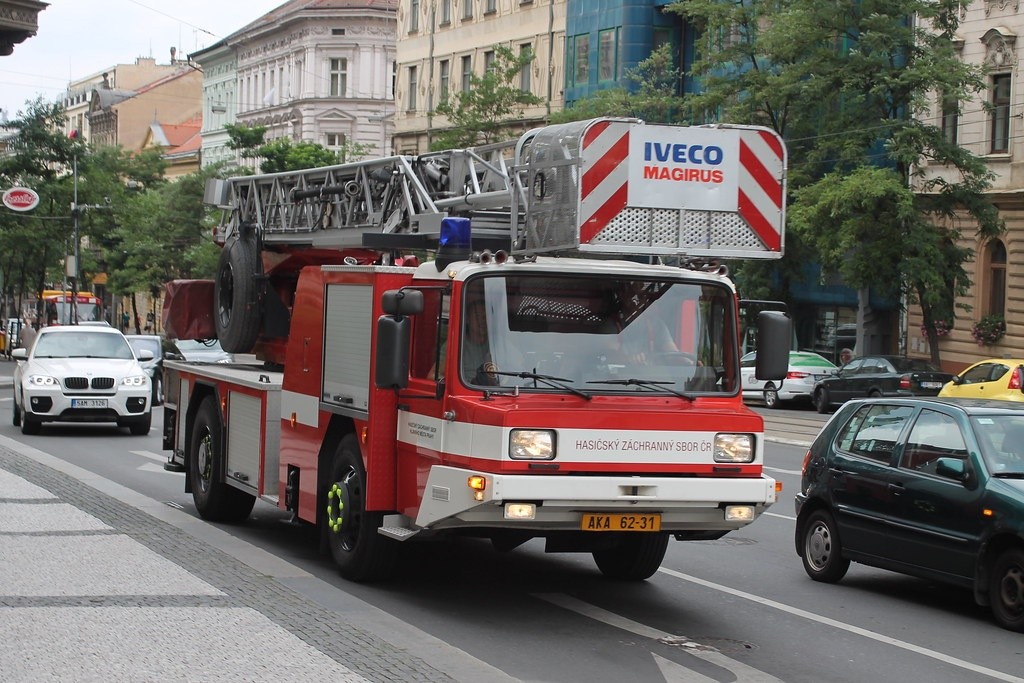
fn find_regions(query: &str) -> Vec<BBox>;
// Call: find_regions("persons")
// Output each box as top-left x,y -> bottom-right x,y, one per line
601,280 -> 704,368
427,301 -> 524,382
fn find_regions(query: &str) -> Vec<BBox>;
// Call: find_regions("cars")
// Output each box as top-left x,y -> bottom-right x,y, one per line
810,354 -> 953,413
73,319 -> 112,327
938,357 -> 1024,403
714,350 -> 841,410
119,335 -> 189,405
11,325 -> 155,435
0,318 -> 28,356
171,335 -> 237,366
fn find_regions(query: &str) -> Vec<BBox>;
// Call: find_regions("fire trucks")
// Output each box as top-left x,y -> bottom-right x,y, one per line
43,289 -> 106,326
150,114 -> 790,588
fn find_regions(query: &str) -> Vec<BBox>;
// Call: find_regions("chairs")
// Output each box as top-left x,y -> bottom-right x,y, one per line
999,424 -> 1024,470
907,428 -> 968,478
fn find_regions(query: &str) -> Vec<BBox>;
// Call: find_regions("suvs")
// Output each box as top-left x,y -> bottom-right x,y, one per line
793,395 -> 1024,632
